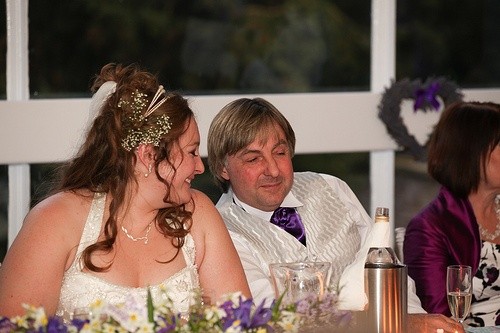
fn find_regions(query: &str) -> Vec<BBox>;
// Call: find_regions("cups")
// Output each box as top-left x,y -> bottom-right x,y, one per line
269,261 -> 331,314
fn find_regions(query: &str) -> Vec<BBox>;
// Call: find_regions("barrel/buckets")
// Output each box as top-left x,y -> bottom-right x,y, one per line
362,267 -> 408,333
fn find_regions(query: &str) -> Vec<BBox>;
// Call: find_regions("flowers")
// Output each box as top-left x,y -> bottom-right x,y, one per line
0,285 -> 354,333
117,87 -> 172,151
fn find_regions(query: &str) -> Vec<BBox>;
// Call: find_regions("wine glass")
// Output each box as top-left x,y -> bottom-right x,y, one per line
447,265 -> 472,326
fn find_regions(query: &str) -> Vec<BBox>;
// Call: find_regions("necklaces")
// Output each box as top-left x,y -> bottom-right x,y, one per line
111,216 -> 156,247
472,193 -> 500,241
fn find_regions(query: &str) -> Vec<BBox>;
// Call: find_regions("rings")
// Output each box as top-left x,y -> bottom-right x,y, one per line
437,329 -> 444,333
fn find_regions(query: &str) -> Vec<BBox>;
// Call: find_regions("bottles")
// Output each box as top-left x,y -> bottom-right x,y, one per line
366,207 -> 398,264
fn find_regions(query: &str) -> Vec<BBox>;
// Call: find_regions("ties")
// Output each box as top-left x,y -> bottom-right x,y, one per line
270,207 -> 306,246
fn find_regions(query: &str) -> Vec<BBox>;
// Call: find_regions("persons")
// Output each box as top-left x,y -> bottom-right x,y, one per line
0,63 -> 256,333
207,98 -> 467,333
403,100 -> 500,333
